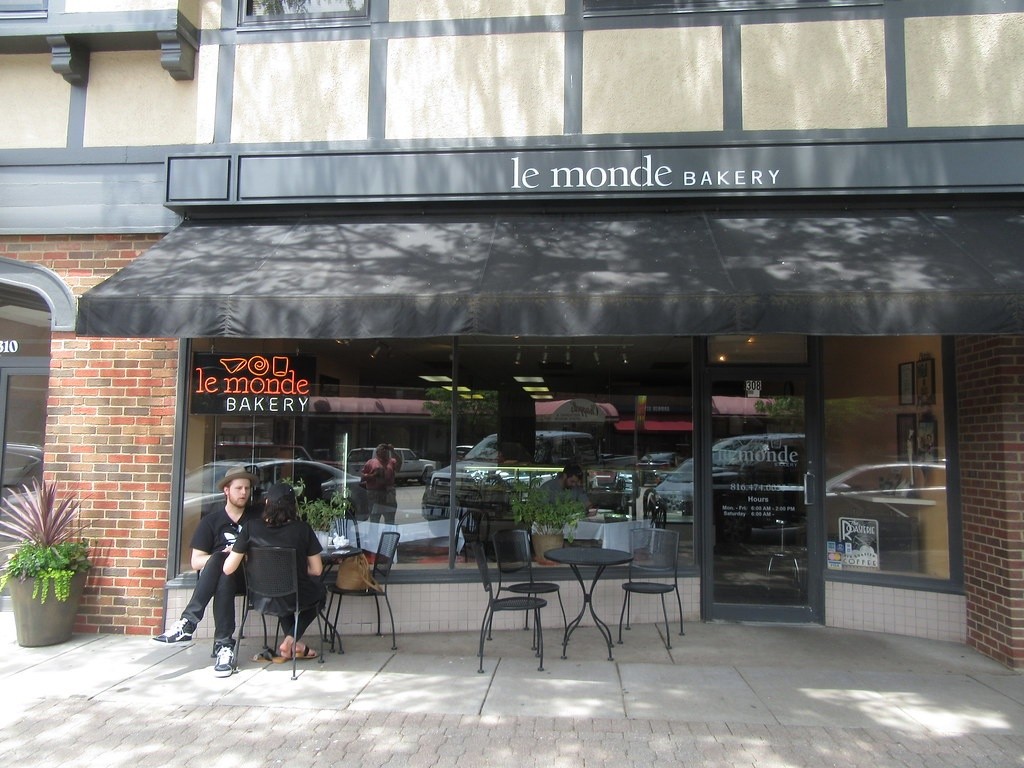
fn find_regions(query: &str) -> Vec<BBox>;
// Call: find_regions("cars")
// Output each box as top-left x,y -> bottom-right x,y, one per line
0,440 -> 45,545
181,457 -> 362,568
825,460 -> 948,548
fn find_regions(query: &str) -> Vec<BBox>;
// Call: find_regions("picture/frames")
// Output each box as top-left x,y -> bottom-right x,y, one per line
898,361 -> 915,404
896,412 -> 918,460
918,419 -> 938,463
916,358 -> 935,406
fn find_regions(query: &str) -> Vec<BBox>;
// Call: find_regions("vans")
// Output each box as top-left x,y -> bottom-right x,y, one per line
650,432 -> 807,554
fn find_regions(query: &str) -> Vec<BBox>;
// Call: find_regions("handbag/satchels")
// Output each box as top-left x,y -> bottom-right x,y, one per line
335,553 -> 384,592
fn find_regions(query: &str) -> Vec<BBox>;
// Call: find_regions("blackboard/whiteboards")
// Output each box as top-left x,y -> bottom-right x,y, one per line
838,516 -> 880,571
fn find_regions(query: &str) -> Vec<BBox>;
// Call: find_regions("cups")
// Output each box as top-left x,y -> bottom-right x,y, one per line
317,531 -> 329,554
328,537 -> 333,545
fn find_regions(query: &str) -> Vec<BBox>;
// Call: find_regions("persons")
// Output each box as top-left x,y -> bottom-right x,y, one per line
222,484 -> 323,663
150,467 -> 259,678
361,444 -> 402,547
539,465 -> 593,510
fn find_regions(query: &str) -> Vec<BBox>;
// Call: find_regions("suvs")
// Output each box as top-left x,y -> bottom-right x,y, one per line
636,450 -> 680,484
347,446 -> 439,485
211,441 -> 314,463
439,444 -> 474,468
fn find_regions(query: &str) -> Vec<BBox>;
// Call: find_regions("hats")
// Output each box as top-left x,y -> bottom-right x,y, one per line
217,466 -> 258,492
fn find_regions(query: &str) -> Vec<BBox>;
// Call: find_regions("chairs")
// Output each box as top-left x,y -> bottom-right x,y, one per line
486,529 -> 570,649
616,529 -> 684,649
473,542 -> 547,673
327,506 -> 360,548
418,490 -> 491,562
210,532 -> 400,680
642,488 -> 666,529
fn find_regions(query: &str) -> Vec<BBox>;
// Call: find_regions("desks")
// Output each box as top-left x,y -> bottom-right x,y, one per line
543,547 -> 635,660
317,546 -> 362,653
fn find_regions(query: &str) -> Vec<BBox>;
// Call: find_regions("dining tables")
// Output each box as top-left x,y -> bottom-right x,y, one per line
335,510 -> 466,563
531,509 -> 654,567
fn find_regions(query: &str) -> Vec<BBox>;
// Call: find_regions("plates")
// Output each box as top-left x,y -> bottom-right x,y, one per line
327,545 -> 334,549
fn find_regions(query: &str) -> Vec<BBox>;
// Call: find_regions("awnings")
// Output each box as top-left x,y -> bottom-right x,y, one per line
614,421 -> 692,431
712,395 -> 778,416
76,210 -> 1024,336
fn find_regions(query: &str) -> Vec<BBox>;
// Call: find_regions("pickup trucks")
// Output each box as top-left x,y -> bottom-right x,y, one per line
421,429 -> 642,542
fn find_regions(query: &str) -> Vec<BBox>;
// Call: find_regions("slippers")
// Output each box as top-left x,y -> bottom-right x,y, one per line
250,648 -> 286,663
281,644 -> 317,659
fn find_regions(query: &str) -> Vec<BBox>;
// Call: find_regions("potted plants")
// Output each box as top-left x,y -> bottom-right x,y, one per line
0,475 -> 100,647
509,469 -> 589,566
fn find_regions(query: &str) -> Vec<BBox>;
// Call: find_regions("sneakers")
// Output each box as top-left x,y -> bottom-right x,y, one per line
213,644 -> 235,676
149,617 -> 195,646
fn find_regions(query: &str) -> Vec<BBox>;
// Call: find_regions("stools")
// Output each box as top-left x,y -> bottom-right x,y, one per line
765,517 -> 801,592
